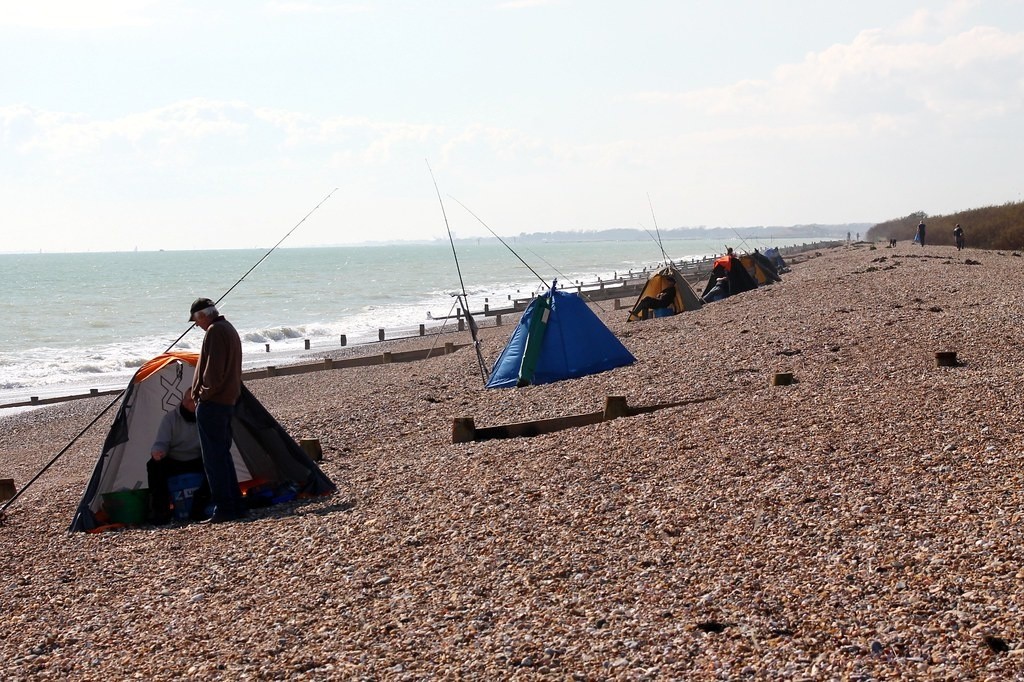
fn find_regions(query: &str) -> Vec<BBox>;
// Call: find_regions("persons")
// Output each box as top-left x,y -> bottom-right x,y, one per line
953,224 -> 964,251
139,386 -> 215,525
700,276 -> 730,303
727,248 -> 737,258
917,221 -> 926,247
188,297 -> 250,522
628,278 -> 676,320
890,238 -> 896,248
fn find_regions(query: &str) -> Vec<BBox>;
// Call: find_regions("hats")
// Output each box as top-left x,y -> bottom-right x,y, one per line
188,298 -> 214,322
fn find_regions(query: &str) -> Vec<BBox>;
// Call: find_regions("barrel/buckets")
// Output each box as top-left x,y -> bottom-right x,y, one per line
99,489 -> 143,525
167,473 -> 202,520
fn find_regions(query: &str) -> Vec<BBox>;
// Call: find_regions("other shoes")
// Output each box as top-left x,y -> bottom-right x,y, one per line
197,517 -> 216,524
629,311 -> 637,316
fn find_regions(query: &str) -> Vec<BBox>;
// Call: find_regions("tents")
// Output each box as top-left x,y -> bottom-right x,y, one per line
764,249 -> 785,271
627,265 -> 702,323
68,353 -> 338,534
484,281 -> 637,390
700,255 -> 758,304
739,253 -> 780,285
